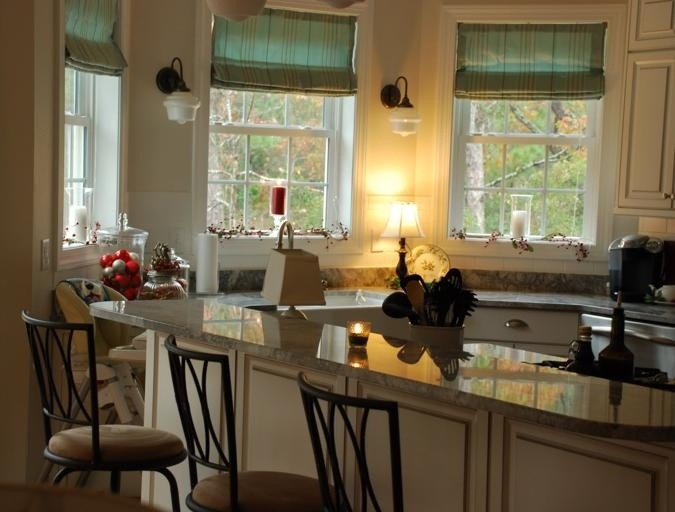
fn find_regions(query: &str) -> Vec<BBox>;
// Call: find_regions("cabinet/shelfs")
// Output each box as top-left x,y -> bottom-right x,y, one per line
461,307 -> 579,358
626,0 -> 675,52
612,49 -> 675,219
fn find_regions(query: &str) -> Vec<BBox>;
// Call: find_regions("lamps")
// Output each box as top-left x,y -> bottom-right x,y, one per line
381,75 -> 422,138
156,55 -> 202,126
381,201 -> 425,283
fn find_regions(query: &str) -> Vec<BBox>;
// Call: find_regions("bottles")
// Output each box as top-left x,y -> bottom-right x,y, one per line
598,308 -> 635,383
564,326 -> 595,376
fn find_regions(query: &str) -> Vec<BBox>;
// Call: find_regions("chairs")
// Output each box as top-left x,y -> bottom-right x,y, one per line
20,308 -> 187,512
52,277 -> 147,429
165,333 -> 346,512
295,369 -> 404,512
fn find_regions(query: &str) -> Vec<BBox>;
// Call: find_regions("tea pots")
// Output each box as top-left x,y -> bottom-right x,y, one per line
649,283 -> 675,302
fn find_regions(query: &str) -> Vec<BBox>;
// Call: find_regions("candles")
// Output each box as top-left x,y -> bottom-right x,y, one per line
66,205 -> 88,243
349,322 -> 368,345
510,209 -> 529,239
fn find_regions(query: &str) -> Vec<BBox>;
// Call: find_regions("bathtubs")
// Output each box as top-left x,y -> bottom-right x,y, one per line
276,288 -> 389,318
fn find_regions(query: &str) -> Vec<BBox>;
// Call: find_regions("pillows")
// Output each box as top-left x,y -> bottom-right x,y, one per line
80,280 -> 109,306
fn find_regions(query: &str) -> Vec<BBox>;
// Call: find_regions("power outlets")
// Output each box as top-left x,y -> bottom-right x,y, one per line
41,238 -> 50,270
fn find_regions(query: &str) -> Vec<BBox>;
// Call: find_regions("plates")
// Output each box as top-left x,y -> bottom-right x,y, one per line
654,299 -> 674,306
407,245 -> 450,284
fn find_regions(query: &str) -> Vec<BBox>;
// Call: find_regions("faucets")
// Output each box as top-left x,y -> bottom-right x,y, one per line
354,288 -> 366,304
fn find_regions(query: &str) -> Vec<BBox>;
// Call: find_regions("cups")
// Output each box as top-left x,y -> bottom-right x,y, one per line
510,194 -> 533,240
345,320 -> 372,351
65,186 -> 93,241
